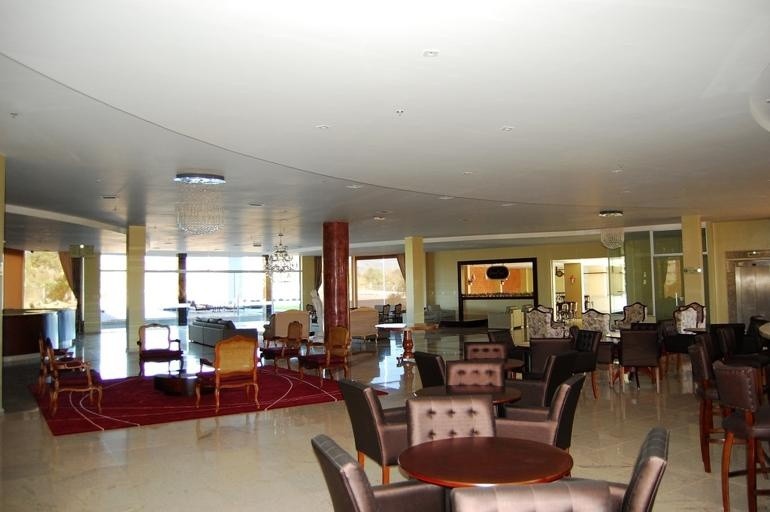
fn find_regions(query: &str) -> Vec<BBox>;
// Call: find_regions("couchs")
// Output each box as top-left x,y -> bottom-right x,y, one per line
262,310 -> 311,352
189,317 -> 258,347
349,307 -> 379,342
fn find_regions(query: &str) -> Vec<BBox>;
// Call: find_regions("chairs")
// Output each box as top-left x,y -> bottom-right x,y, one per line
530,337 -> 571,378
672,302 -> 706,335
298,325 -> 352,388
391,304 -> 403,322
527,308 -> 557,340
507,355 -> 557,408
689,345 -> 770,472
582,309 -> 611,342
337,376 -> 409,485
533,304 -> 566,329
570,325 -> 616,389
614,302 -> 647,330
498,375 -> 587,450
488,329 -> 528,360
446,360 -> 505,387
194,336 -> 259,414
379,304 -> 391,323
414,350 -> 447,389
46,346 -> 103,417
137,323 -> 184,381
310,433 -> 444,512
261,321 -> 304,373
718,327 -> 767,406
464,342 -> 508,362
695,333 -> 758,428
448,479 -> 612,512
406,394 -> 498,445
559,330 -> 602,399
658,318 -> 701,375
611,424 -> 672,512
716,367 -> 770,512
38,338 -> 76,386
619,330 -> 661,394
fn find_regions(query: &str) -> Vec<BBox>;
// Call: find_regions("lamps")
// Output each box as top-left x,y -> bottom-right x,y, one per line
264,233 -> 298,276
599,210 -> 625,249
173,172 -> 226,236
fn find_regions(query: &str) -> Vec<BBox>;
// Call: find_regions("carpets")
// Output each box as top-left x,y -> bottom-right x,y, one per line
28,365 -> 388,425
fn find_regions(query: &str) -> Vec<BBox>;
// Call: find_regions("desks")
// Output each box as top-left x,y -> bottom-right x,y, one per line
376,321 -> 439,368
398,436 -> 575,487
504,358 -> 524,370
415,385 -> 522,417
